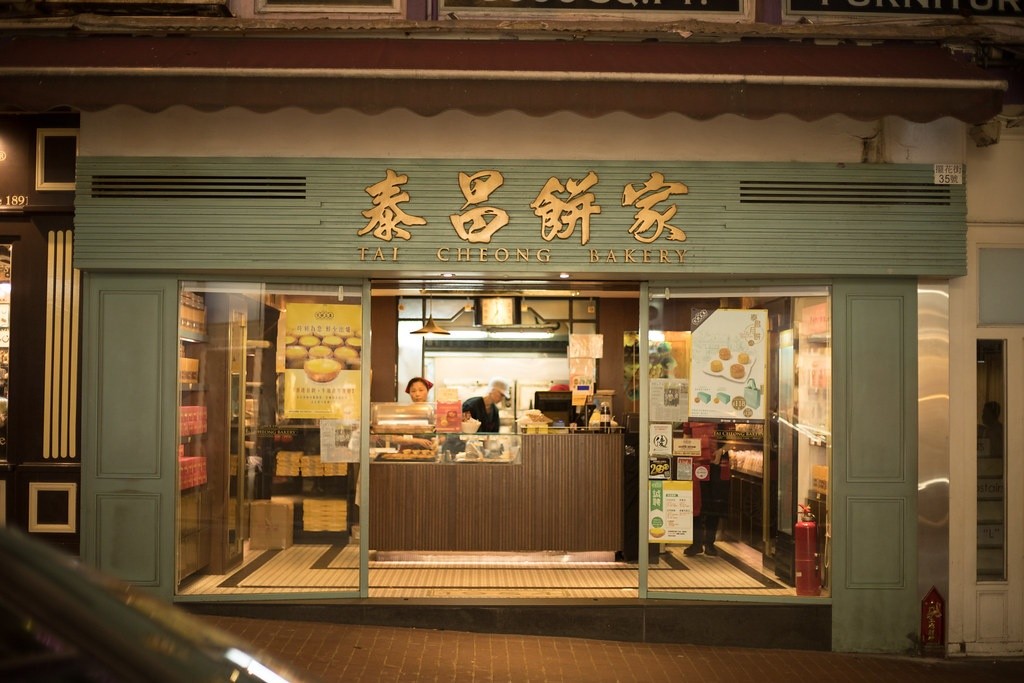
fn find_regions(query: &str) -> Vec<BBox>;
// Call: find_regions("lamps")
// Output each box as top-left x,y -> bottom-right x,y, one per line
410,298 -> 451,335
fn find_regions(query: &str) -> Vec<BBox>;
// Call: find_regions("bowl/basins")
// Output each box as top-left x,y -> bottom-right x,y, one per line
461,422 -> 481,433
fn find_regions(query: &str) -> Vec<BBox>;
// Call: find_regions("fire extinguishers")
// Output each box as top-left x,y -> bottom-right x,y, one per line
794,503 -> 821,597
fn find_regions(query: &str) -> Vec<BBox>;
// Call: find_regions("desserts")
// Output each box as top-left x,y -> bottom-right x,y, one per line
738,353 -> 749,364
720,349 -> 731,360
711,360 -> 722,372
731,364 -> 744,378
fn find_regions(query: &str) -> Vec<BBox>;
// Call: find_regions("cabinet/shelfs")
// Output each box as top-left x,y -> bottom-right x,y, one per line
245,340 -> 353,544
724,469 -> 778,554
179,327 -> 210,580
371,432 -> 625,552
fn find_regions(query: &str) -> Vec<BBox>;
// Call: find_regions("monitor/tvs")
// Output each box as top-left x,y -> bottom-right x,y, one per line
534,391 -> 572,427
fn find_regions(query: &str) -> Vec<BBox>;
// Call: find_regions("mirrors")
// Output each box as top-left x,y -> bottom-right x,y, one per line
229,372 -> 239,551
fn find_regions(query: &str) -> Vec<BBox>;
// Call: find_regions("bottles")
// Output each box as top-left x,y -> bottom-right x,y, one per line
600,402 -> 611,434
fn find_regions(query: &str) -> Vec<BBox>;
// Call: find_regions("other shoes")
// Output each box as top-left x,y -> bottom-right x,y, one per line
684,541 -> 703,555
705,541 -> 719,556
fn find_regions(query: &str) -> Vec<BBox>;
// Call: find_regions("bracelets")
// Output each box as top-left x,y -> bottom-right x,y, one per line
720,448 -> 725,455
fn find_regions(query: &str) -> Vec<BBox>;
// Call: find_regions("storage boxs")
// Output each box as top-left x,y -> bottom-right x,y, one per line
249,499 -> 294,549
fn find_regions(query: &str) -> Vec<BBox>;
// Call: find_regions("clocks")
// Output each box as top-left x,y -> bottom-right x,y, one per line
474,297 -> 522,326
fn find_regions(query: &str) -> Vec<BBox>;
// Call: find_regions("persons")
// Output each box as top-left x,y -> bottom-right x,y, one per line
441,378 -> 511,455
405,377 -> 433,402
683,422 -> 737,556
981,402 -> 1003,459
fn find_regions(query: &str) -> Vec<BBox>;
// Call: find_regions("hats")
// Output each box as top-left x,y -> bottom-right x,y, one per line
405,377 -> 433,393
490,380 -> 510,398
